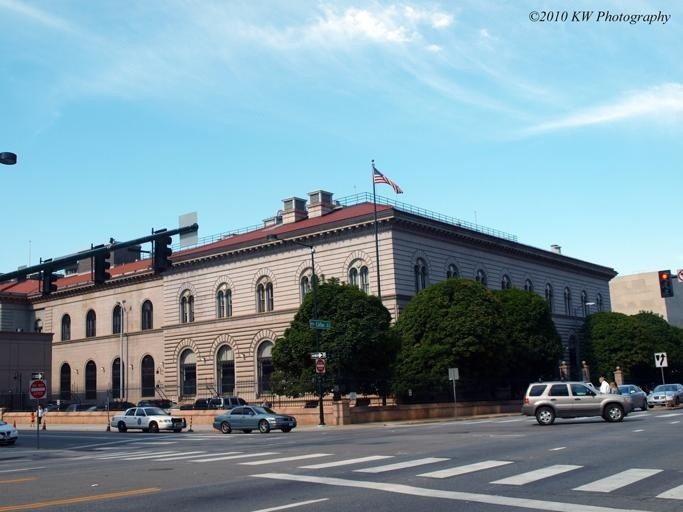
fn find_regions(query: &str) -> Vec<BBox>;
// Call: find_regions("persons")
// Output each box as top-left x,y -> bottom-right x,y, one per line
599,377 -> 618,394
262,400 -> 270,408
36,405 -> 43,424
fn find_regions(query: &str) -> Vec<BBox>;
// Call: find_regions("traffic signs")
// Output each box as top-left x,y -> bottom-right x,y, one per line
310,351 -> 326,359
32,372 -> 43,380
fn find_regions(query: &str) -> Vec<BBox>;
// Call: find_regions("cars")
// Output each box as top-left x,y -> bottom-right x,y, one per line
0,421 -> 18,445
520,381 -> 683,426
47,397 -> 297,434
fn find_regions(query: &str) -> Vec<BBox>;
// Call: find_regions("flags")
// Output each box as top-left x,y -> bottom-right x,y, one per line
374,167 -> 403,194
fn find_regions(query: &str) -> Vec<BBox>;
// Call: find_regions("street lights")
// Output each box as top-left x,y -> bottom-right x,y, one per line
116,299 -> 126,402
14,372 -> 22,410
574,302 -> 595,373
267,234 -> 325,425
1,152 -> 17,165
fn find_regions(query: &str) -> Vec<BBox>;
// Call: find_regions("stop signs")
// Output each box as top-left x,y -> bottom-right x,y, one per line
29,381 -> 47,399
315,360 -> 325,375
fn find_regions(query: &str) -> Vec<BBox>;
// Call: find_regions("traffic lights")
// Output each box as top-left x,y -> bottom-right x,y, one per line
42,259 -> 58,296
95,244 -> 111,284
154,236 -> 172,272
658,270 -> 674,297
